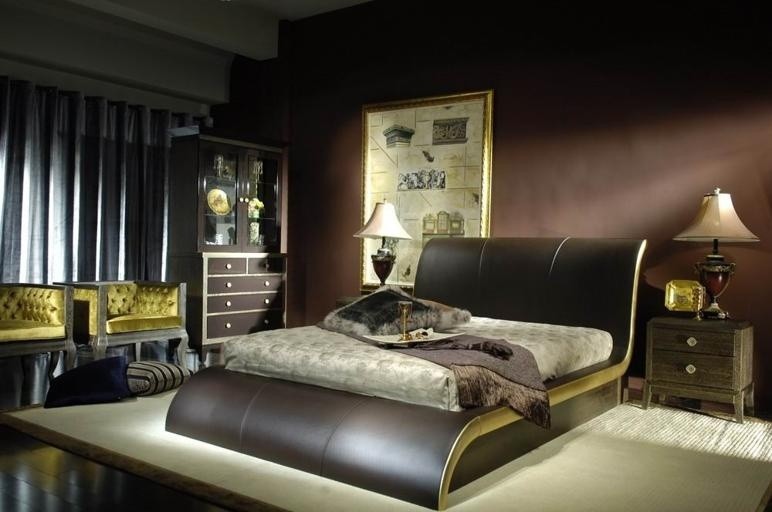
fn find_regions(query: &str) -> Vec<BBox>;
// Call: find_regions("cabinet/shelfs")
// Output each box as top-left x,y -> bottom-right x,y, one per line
167,123 -> 285,371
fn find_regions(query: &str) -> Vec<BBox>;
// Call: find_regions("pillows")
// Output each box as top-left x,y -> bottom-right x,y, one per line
314,287 -> 469,346
44,355 -> 198,410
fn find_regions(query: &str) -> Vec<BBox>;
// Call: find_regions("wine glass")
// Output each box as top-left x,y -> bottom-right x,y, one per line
396,300 -> 413,342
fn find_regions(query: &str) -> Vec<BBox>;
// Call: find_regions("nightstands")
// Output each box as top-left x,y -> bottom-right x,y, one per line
638,315 -> 758,426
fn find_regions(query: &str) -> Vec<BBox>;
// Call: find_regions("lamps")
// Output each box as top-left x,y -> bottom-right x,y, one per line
671,188 -> 762,321
353,199 -> 413,290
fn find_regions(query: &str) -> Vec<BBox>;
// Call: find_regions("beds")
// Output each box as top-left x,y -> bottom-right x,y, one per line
166,237 -> 651,511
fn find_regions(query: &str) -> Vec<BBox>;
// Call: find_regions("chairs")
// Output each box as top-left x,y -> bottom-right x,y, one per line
0,278 -> 189,378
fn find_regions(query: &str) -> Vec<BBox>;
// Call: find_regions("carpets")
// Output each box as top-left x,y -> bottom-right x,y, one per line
0,388 -> 772,512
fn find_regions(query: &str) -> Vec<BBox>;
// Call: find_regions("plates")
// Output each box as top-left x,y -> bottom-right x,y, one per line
360,327 -> 466,344
206,187 -> 232,216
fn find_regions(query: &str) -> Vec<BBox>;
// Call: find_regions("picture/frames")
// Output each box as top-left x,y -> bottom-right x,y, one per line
360,88 -> 497,291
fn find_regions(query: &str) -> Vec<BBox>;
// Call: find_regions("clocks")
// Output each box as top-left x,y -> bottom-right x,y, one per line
661,279 -> 707,315
